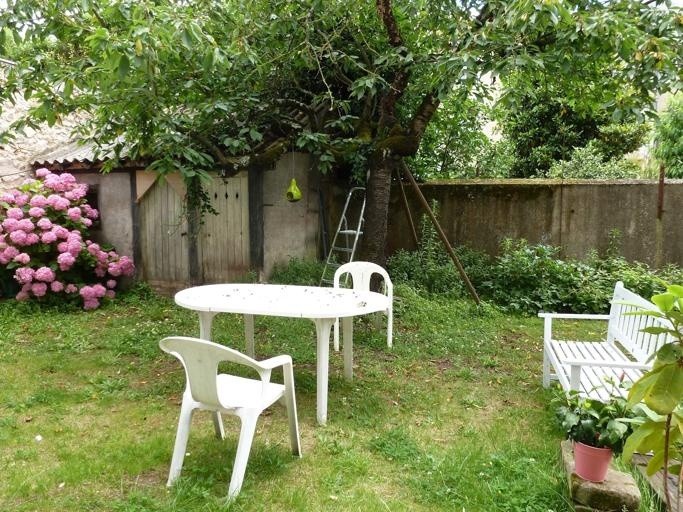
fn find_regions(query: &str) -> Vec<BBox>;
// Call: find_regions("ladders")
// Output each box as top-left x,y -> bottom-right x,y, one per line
319,187 -> 368,288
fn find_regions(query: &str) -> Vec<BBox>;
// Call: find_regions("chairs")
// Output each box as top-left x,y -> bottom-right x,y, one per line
158,337 -> 302,497
332,262 -> 392,351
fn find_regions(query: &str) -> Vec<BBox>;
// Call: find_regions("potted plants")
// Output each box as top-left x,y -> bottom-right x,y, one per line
551,374 -> 637,482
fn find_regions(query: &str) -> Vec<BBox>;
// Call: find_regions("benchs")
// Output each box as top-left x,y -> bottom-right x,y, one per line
536,281 -> 680,405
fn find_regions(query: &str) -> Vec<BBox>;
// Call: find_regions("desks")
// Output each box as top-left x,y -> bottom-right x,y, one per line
174,283 -> 389,425
